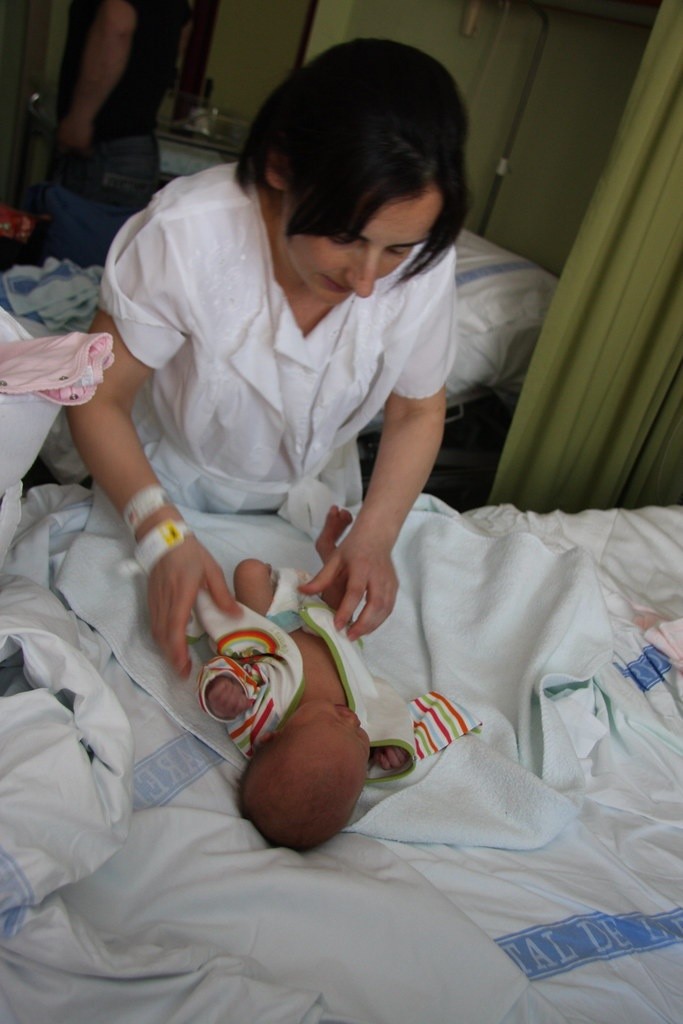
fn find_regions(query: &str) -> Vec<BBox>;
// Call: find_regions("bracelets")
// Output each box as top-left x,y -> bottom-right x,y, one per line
123,482 -> 177,538
131,515 -> 191,576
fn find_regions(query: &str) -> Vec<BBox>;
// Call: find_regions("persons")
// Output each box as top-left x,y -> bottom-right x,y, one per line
47,0 -> 476,677
198,504 -> 416,850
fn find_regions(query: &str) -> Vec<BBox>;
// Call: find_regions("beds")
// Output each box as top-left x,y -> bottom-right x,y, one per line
0,503 -> 683,1024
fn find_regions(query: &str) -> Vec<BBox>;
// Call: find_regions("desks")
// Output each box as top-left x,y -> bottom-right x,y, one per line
156,90 -> 250,180
